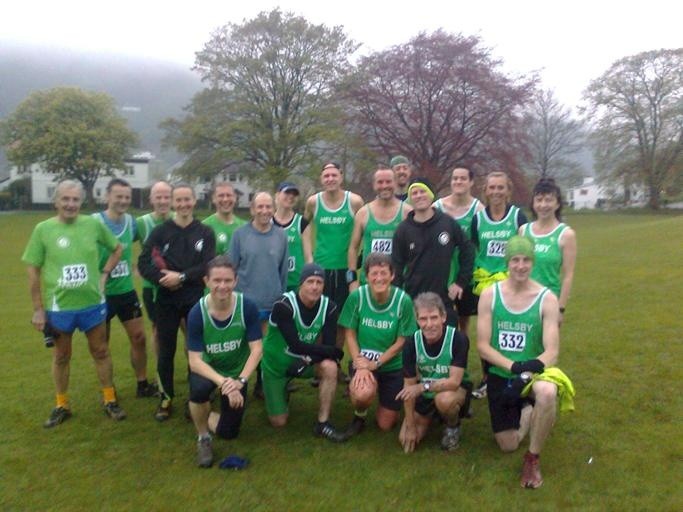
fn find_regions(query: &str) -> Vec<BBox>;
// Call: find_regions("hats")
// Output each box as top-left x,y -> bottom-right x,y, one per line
407,177 -> 434,201
390,155 -> 407,167
278,181 -> 300,196
300,263 -> 326,286
319,160 -> 340,175
505,234 -> 535,268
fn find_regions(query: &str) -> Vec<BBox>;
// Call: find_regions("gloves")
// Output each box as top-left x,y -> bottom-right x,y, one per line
511,359 -> 545,374
286,357 -> 309,378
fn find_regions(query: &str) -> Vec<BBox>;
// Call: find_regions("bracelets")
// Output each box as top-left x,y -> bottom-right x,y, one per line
375,359 -> 381,368
520,371 -> 530,383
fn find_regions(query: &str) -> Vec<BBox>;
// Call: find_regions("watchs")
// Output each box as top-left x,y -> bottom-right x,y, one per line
235,376 -> 247,385
423,381 -> 431,391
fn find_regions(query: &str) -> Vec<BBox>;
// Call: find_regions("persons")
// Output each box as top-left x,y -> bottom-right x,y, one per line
91,180 -> 162,398
134,181 -> 176,327
183,255 -> 264,468
258,263 -> 349,442
477,236 -> 561,489
202,155 -> 576,400
21,177 -> 128,427
137,183 -> 215,423
337,251 -> 417,439
393,290 -> 469,454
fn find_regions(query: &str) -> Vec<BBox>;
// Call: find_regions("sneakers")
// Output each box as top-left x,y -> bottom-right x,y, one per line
521,452 -> 543,488
470,378 -> 488,399
45,382 -> 171,427
313,421 -> 347,444
196,432 -> 214,468
345,417 -> 365,438
441,426 -> 461,452
